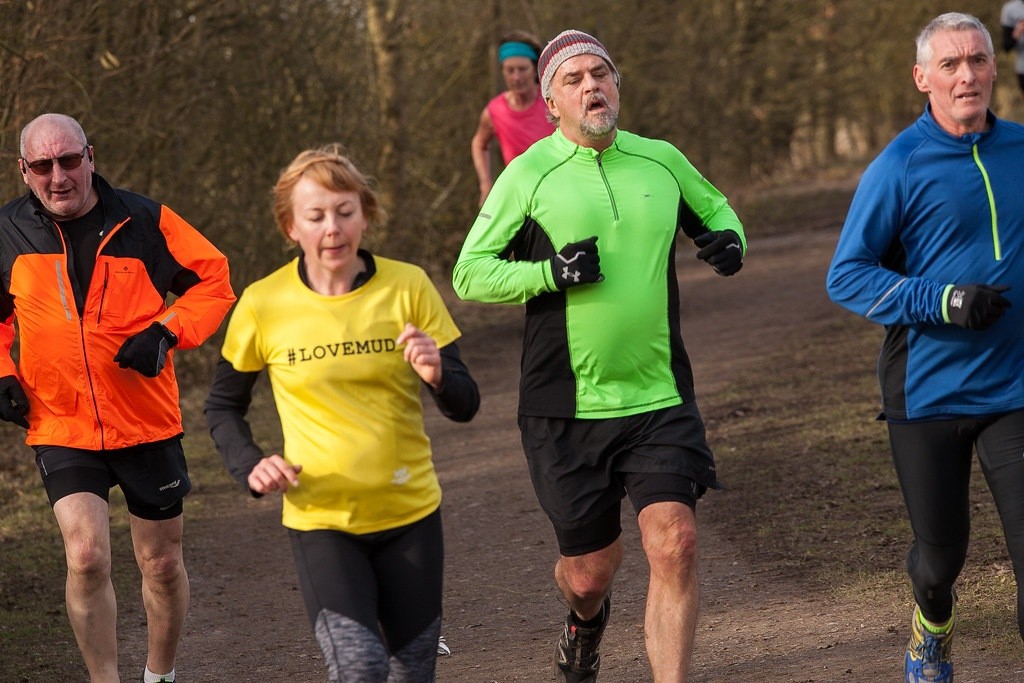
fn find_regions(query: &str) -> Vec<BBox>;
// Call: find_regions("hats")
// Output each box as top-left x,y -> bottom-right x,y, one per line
538,29 -> 620,103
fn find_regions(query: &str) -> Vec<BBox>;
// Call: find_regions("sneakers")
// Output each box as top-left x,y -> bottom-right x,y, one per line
902,585 -> 959,683
553,589 -> 612,683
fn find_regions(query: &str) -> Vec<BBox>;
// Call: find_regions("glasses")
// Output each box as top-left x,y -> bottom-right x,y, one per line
22,144 -> 90,175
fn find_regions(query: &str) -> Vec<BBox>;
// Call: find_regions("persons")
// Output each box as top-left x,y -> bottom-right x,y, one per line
471,28 -> 556,212
824,11 -> 1024,683
451,30 -> 747,683
0,113 -> 237,683
998,0 -> 1024,96
203,140 -> 478,682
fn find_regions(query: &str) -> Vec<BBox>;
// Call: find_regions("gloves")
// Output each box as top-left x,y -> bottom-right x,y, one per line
550,236 -> 605,289
694,229 -> 745,277
0,375 -> 30,429
113,322 -> 179,377
948,283 -> 1013,332
